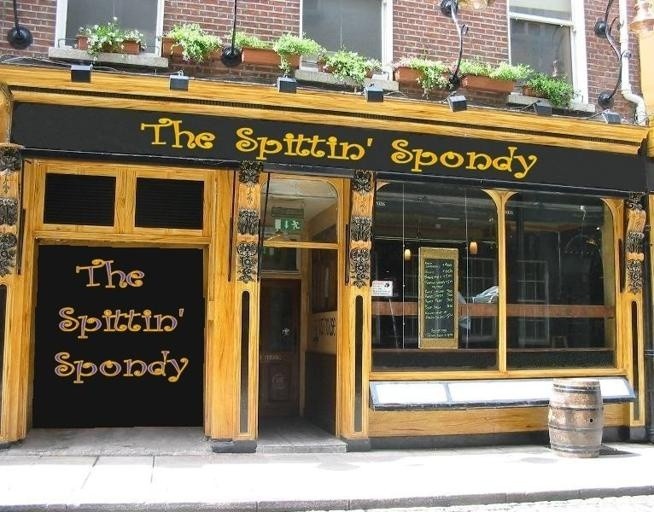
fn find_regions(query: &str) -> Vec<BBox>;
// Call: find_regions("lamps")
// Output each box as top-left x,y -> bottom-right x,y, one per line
69,63 -> 92,83
363,87 -> 384,103
276,77 -> 297,94
437,0 -> 496,94
220,1 -> 243,69
520,98 -> 553,115
600,107 -> 621,127
168,69 -> 189,92
447,96 -> 470,112
591,0 -> 654,111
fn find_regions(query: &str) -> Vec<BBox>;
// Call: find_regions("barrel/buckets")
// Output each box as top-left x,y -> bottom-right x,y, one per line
548,380 -> 604,458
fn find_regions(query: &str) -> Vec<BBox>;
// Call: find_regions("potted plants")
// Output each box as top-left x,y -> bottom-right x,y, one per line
453,57 -> 535,98
77,15 -> 146,62
521,73 -> 577,112
234,29 -> 326,77
157,23 -> 229,65
316,41 -> 384,90
391,56 -> 453,100
